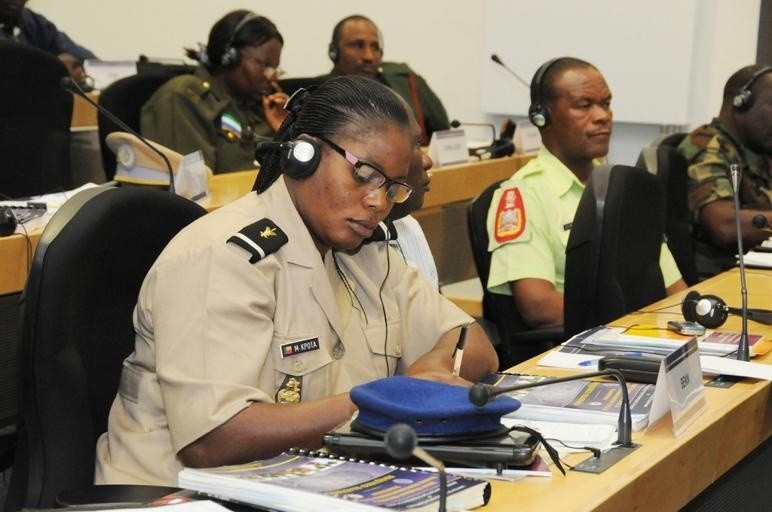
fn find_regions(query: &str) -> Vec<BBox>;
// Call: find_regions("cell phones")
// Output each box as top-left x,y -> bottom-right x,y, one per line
665,316 -> 707,337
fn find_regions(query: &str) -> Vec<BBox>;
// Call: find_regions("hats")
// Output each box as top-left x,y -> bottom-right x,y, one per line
349,375 -> 521,446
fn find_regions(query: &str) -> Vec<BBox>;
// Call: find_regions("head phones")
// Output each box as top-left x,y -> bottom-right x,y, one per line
683,288 -> 772,329
527,56 -> 560,129
220,11 -> 265,72
251,131 -> 325,181
329,14 -> 387,66
732,67 -> 772,113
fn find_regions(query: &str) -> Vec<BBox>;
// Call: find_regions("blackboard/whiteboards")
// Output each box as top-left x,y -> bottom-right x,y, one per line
24,0 -> 772,127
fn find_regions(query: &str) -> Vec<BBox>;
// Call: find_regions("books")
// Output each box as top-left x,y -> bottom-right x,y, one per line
535,324 -> 767,377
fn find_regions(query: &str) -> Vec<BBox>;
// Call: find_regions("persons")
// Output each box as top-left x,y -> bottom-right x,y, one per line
138,9 -> 290,175
675,63 -> 772,287
1,0 -> 103,81
486,55 -> 687,352
309,16 -> 451,148
388,88 -> 442,294
90,72 -> 500,511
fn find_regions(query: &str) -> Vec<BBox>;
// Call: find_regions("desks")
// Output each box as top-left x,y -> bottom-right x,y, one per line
72,91 -> 98,129
410,138 -> 539,318
0,228 -> 45,294
204,170 -> 261,213
152,270 -> 770,511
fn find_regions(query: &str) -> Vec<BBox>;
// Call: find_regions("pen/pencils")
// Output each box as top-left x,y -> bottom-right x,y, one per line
576,352 -> 641,366
450,324 -> 467,377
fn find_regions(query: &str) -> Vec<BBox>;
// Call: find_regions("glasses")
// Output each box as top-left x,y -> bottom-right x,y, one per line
307,132 -> 414,204
240,49 -> 285,81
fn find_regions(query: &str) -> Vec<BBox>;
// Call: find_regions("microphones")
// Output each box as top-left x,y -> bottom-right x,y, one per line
467,366 -> 634,448
751,212 -> 772,234
728,154 -> 753,362
55,70 -> 177,197
382,420 -> 456,511
450,117 -> 517,158
489,49 -> 534,92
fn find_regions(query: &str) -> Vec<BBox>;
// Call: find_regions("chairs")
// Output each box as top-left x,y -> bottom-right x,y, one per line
279,77 -> 314,98
96,71 -> 177,182
465,132 -> 697,371
16,184 -> 208,509
